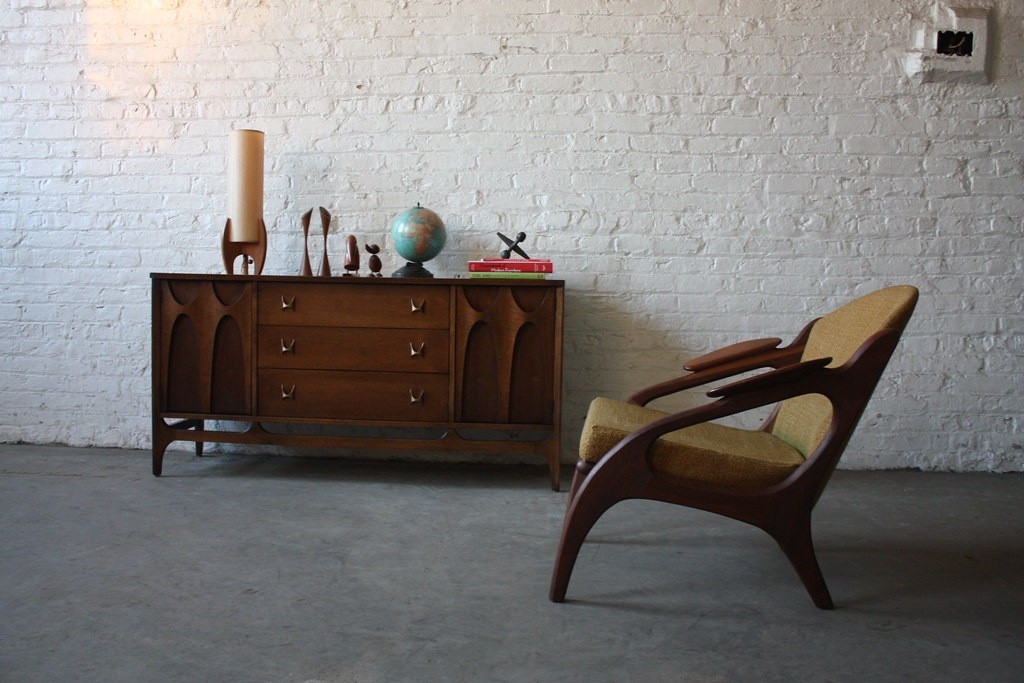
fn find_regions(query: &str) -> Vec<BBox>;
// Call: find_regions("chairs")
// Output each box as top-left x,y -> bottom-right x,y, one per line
549,283 -> 919,611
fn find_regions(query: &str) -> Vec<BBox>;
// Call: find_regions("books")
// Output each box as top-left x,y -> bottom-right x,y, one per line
466,257 -> 552,279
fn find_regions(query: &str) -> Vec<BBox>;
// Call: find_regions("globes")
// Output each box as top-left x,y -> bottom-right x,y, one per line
390,201 -> 448,279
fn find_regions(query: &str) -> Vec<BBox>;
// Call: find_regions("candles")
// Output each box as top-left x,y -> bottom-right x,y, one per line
227,129 -> 265,242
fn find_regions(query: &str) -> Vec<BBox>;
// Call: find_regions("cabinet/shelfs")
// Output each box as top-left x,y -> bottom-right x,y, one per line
150,271 -> 567,491
